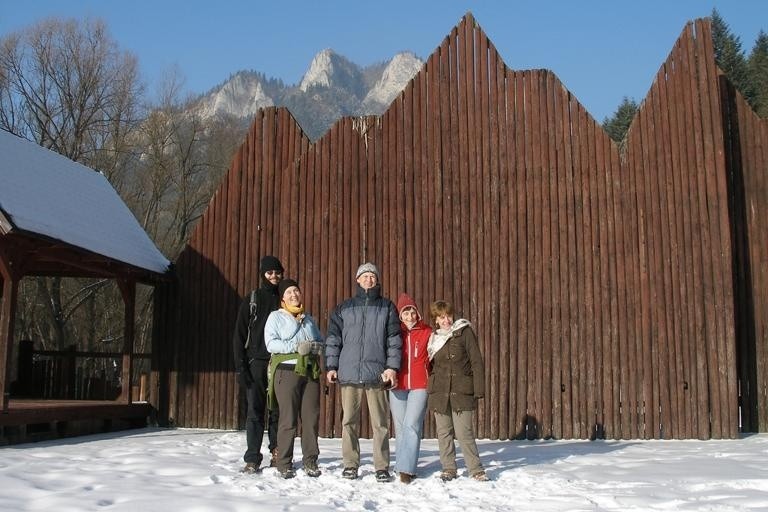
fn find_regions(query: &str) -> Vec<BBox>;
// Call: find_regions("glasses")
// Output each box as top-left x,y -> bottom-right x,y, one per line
267,271 -> 280,275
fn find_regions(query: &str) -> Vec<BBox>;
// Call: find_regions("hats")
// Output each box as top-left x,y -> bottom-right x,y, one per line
261,256 -> 284,273
278,279 -> 301,299
355,263 -> 380,282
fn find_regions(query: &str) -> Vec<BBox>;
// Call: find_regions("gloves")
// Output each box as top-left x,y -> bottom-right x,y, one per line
296,341 -> 323,355
239,365 -> 254,389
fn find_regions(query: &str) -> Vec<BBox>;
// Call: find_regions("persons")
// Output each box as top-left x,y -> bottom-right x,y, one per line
425,299 -> 492,483
323,263 -> 402,483
263,276 -> 325,479
386,291 -> 433,483
230,253 -> 287,478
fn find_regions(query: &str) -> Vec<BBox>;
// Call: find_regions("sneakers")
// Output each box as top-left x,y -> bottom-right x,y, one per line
473,471 -> 491,481
440,470 -> 456,481
244,447 -> 321,478
342,467 -> 417,484
